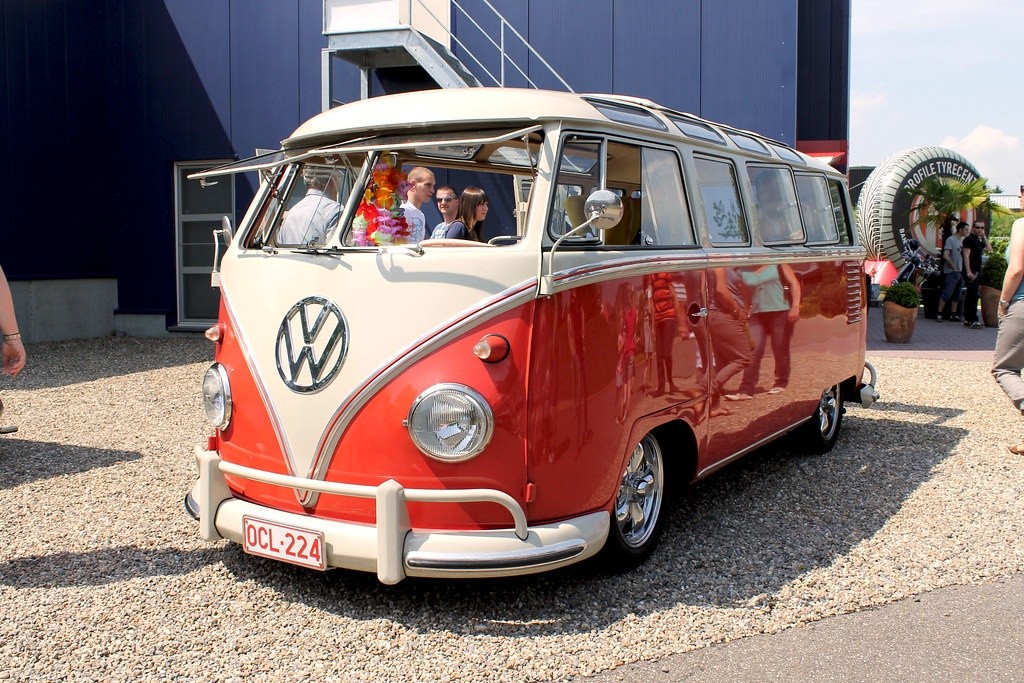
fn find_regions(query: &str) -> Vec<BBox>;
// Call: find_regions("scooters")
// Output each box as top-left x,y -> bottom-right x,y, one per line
891,239 -> 942,304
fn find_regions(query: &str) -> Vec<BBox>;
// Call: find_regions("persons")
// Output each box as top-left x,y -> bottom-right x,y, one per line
725,264 -> 801,400
398,167 -> 435,243
443,185 -> 489,244
0,266 -> 26,415
647,272 -> 686,397
275,161 -> 354,246
560,284 -> 605,459
936,222 -> 970,322
699,267 -> 752,417
961,219 -> 992,328
614,284 -> 638,422
429,186 -> 458,240
991,179 -> 1024,455
642,274 -> 655,388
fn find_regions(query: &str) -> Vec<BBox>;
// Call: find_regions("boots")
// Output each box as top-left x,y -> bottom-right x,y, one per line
652,360 -> 677,400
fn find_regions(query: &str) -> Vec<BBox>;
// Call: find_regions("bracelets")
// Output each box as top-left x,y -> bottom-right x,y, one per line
3,332 -> 21,339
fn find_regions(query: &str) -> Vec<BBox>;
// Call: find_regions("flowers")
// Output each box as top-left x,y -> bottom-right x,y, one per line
871,268 -> 877,284
352,150 -> 417,246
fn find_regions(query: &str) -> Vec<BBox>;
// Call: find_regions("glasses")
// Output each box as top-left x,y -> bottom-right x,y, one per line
974,226 -> 985,229
436,197 -> 459,202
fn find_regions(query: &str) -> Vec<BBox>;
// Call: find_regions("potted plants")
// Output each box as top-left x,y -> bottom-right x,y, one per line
901,175 -> 1015,319
879,285 -> 887,300
883,282 -> 919,343
979,254 -> 1008,327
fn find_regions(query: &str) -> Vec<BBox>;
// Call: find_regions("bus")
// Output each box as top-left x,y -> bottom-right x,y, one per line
183,87 -> 881,585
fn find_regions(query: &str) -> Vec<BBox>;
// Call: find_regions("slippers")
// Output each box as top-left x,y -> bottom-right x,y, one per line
1009,441 -> 1024,455
711,408 -> 734,419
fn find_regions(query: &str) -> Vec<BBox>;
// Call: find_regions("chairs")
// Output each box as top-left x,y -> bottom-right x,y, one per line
604,196 -> 641,245
565,196 -> 592,236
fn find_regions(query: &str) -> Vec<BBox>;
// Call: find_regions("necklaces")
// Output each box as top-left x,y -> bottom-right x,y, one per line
354,150 -> 417,246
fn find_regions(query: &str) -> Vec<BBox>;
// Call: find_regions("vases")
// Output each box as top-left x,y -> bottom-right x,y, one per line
870,284 -> 880,299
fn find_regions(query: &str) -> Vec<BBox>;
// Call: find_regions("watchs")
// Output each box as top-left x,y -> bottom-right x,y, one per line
999,300 -> 1009,308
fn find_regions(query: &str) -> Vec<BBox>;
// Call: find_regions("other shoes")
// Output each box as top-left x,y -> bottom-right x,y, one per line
949,315 -> 964,322
937,315 -> 943,322
964,321 -> 985,329
725,394 -> 753,401
768,387 -> 785,393
